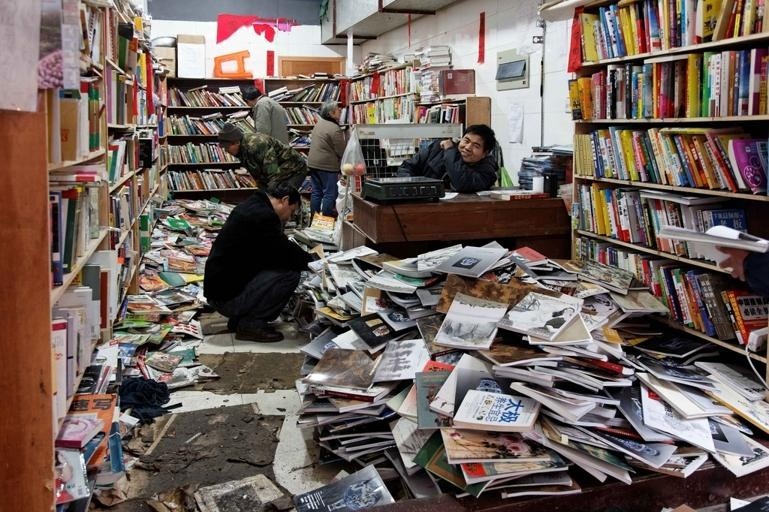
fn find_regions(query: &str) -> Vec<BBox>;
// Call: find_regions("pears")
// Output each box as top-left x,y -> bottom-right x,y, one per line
342,164 -> 354,175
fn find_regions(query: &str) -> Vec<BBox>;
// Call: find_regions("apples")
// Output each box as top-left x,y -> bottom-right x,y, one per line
355,164 -> 366,176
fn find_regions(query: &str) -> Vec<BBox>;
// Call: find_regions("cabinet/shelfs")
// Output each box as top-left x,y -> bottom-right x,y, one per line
0,1 -> 169,512
564,1 -> 767,376
161,68 -> 495,193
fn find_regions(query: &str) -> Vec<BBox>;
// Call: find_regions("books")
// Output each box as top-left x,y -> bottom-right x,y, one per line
489,0 -> 769,273
280,213 -> 768,511
267,44 -> 476,211
2,1 -> 260,512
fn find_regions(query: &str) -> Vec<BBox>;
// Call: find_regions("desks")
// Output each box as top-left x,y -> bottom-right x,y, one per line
348,190 -> 569,261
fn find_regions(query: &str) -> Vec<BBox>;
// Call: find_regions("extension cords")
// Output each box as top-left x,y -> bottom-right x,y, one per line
748,327 -> 768,352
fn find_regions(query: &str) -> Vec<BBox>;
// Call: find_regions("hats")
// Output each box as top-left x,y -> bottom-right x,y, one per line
218,122 -> 243,142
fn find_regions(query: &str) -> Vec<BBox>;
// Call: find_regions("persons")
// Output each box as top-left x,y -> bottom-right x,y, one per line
388,124 -> 500,194
308,100 -> 351,228
242,85 -> 290,145
218,122 -> 308,228
203,182 -> 316,343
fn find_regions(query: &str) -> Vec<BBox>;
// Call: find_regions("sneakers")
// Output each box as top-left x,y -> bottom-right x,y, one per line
227,319 -> 284,343
